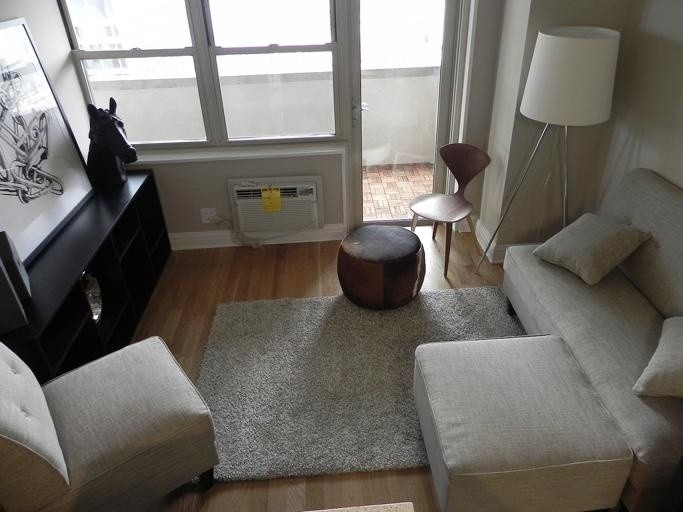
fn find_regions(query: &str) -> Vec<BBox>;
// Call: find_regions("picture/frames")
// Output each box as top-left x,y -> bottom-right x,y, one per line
0,16 -> 94,271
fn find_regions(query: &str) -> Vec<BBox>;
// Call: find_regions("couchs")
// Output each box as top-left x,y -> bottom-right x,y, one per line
0,334 -> 221,512
411,333 -> 635,511
503,166 -> 683,512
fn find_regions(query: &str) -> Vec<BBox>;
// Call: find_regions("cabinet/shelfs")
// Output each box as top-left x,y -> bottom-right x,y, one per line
0,168 -> 174,381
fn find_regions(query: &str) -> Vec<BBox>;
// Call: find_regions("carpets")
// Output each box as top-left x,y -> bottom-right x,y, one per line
191,283 -> 528,484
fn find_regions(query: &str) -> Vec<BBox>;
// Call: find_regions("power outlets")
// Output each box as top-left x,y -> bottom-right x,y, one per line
198,206 -> 217,225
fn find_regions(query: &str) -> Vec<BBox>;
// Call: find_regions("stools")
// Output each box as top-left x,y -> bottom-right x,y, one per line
337,222 -> 426,311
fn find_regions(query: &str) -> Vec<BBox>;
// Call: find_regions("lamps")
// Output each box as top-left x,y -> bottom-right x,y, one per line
474,25 -> 621,274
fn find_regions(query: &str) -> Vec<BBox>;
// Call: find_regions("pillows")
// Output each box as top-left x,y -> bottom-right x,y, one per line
632,315 -> 682,400
532,211 -> 652,288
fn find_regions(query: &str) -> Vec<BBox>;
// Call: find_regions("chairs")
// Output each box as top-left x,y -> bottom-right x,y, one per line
407,142 -> 493,279
